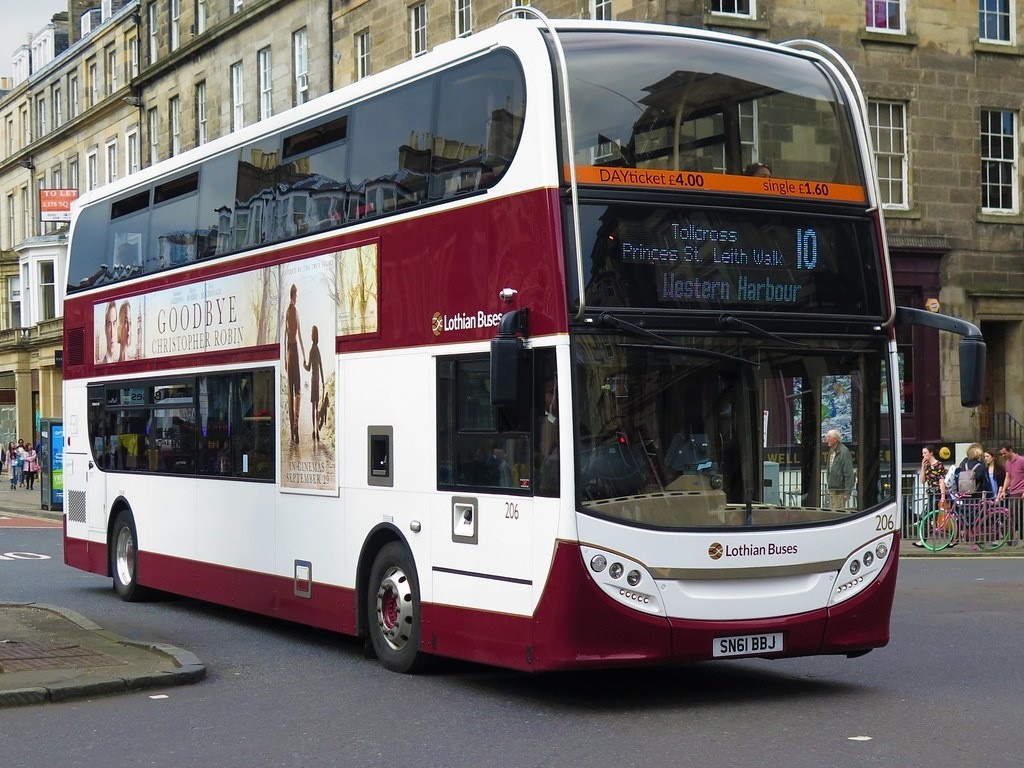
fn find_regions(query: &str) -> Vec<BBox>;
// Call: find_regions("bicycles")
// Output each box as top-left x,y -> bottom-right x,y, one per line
918,489 -> 1012,552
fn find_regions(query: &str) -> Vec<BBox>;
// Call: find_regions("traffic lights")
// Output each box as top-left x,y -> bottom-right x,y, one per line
615,430 -> 639,469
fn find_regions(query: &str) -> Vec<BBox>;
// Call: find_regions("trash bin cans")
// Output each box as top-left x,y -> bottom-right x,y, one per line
764,461 -> 780,506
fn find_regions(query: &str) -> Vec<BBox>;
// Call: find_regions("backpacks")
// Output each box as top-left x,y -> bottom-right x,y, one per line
958,463 -> 981,494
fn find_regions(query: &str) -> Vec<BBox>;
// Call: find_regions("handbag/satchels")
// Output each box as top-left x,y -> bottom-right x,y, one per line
15,454 -> 22,466
1,447 -> 6,461
937,503 -> 952,532
944,464 -> 963,507
32,464 -> 40,472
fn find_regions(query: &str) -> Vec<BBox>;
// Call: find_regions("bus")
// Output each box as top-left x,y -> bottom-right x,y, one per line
63,5 -> 991,678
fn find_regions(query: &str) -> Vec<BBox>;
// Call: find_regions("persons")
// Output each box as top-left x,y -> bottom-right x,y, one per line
827,429 -> 854,509
912,442 -> 1024,548
490,449 -> 511,486
97,301 -> 132,364
744,163 -> 772,178
0,436 -> 47,490
215,450 -> 233,474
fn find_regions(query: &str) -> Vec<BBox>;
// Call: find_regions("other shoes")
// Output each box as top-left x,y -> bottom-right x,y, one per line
0,480 -> 1,482
946,540 -> 959,549
993,540 -> 1006,547
912,543 -> 926,549
312,432 -> 315,439
317,429 -> 319,440
291,427 -> 299,444
